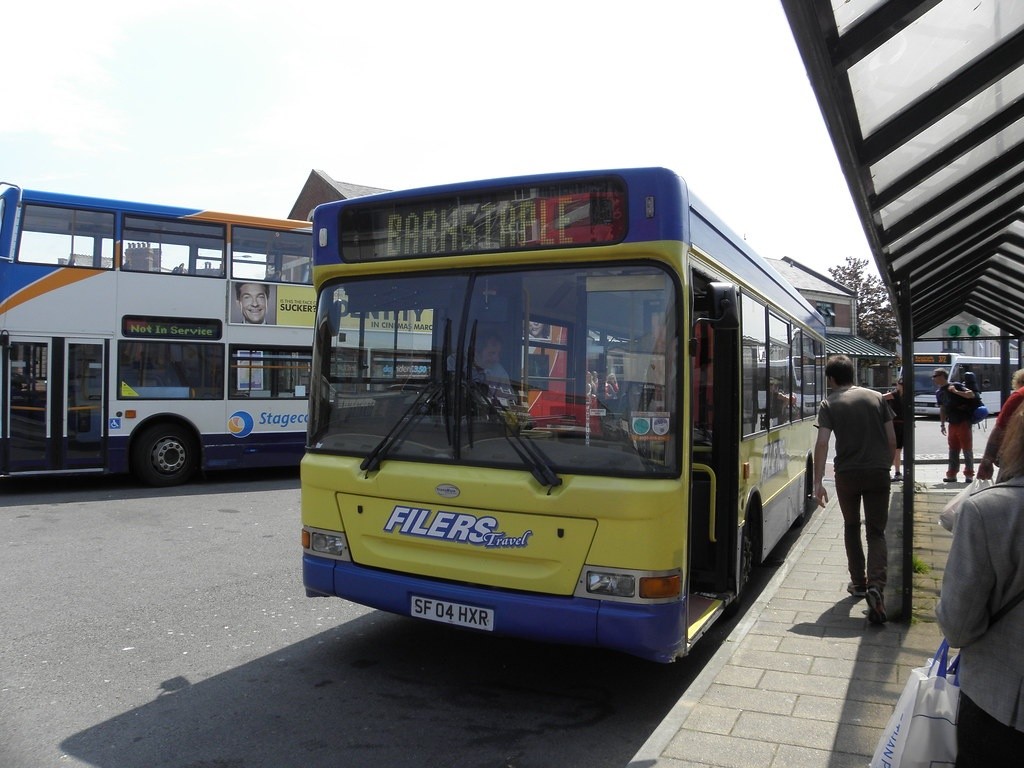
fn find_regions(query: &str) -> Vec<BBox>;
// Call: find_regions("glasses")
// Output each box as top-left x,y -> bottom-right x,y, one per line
898,382 -> 903,385
932,374 -> 940,378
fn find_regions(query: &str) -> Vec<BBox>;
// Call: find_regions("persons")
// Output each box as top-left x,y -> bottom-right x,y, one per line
234,282 -> 270,325
934,399 -> 1024,768
769,376 -> 799,426
585,370 -> 620,409
447,332 -> 520,429
931,368 -> 981,483
814,354 -> 897,625
881,377 -> 906,482
976,369 -> 1024,481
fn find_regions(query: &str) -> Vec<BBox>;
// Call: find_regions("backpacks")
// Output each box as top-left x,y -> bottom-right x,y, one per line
950,372 -> 989,424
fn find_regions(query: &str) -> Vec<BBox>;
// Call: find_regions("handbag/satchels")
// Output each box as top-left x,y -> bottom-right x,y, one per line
871,638 -> 960,768
937,476 -> 995,533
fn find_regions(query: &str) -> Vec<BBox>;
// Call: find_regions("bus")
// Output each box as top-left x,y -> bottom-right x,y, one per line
298,161 -> 832,664
0,184 -> 319,490
897,351 -> 1024,419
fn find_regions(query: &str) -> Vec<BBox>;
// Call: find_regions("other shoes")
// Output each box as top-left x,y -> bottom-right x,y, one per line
865,587 -> 888,623
966,475 -> 973,482
943,477 -> 958,482
894,473 -> 904,480
847,581 -> 866,596
